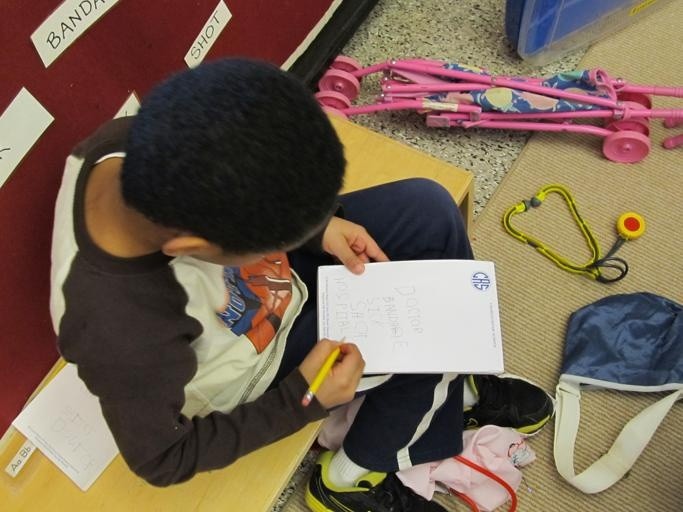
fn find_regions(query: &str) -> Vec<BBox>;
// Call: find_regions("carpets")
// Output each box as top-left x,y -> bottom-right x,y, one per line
278,0 -> 682,511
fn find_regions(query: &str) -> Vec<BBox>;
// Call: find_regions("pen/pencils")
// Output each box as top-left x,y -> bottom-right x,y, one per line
301,334 -> 347,408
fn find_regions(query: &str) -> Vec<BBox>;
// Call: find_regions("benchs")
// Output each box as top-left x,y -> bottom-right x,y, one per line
0,114 -> 475,511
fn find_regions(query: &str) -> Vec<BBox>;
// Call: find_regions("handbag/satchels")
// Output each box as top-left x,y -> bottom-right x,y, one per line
552,292 -> 682,495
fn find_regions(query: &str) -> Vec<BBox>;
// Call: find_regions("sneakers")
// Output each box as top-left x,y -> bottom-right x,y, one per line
305,451 -> 450,512
462,374 -> 554,434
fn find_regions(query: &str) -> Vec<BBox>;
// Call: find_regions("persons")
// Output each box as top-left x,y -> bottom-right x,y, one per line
49,55 -> 556,511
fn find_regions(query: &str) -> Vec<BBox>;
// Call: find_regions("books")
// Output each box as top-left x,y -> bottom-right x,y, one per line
316,258 -> 505,377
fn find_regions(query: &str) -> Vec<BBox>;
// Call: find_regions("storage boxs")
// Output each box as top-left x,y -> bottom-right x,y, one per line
504,0 -> 670,65
